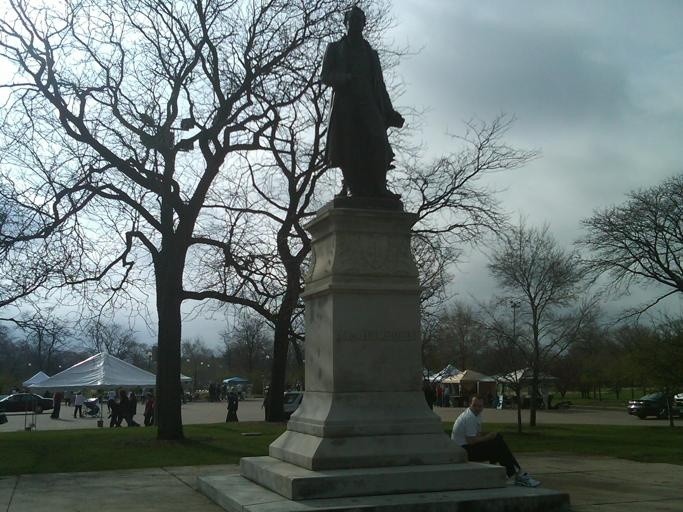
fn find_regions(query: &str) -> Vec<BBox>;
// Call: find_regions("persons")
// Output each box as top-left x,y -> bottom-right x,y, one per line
10,385 -> 18,395
320,5 -> 406,198
208,381 -> 254,423
423,380 -> 496,411
452,394 -> 542,487
261,381 -> 302,407
50,387 -> 155,428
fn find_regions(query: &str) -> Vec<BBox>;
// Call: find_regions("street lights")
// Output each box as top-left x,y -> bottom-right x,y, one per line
510,298 -> 524,372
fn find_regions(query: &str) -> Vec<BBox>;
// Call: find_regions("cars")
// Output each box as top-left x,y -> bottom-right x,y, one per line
627,392 -> 683,420
0,392 -> 54,414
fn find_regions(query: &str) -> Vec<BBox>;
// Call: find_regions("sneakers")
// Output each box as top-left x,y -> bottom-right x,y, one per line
512,473 -> 541,487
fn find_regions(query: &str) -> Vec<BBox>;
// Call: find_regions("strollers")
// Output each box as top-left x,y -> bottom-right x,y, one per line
82,398 -> 100,416
